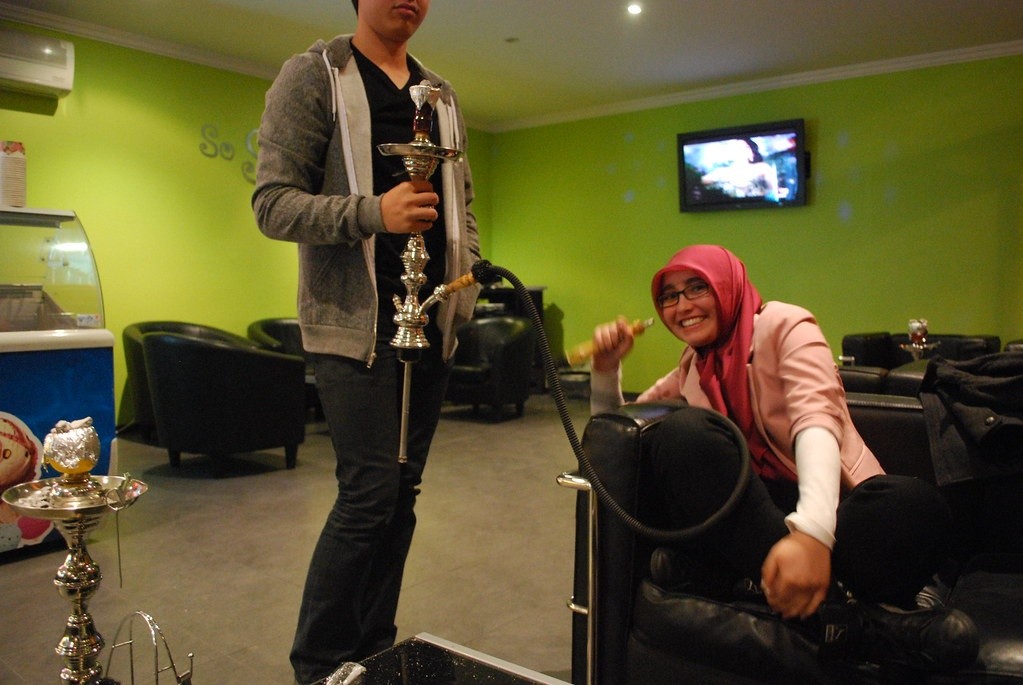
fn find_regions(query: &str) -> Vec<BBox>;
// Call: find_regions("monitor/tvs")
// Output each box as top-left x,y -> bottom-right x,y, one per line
677,118 -> 808,212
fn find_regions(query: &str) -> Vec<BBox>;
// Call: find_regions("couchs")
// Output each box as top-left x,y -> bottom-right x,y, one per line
557,331 -> 1023,685
116,303 -> 530,471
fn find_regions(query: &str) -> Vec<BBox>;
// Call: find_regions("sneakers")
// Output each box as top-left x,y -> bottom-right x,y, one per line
650,547 -> 739,602
823,603 -> 978,672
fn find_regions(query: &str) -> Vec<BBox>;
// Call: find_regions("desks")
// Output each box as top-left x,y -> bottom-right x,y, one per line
309,631 -> 573,685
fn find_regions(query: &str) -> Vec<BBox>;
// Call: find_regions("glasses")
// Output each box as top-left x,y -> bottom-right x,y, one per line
658,282 -> 709,308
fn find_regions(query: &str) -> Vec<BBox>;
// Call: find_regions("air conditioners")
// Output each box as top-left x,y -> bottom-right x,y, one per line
0,25 -> 76,99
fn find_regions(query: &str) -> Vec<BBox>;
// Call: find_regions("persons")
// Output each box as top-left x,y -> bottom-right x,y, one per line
587,243 -> 953,625
251,0 -> 485,685
